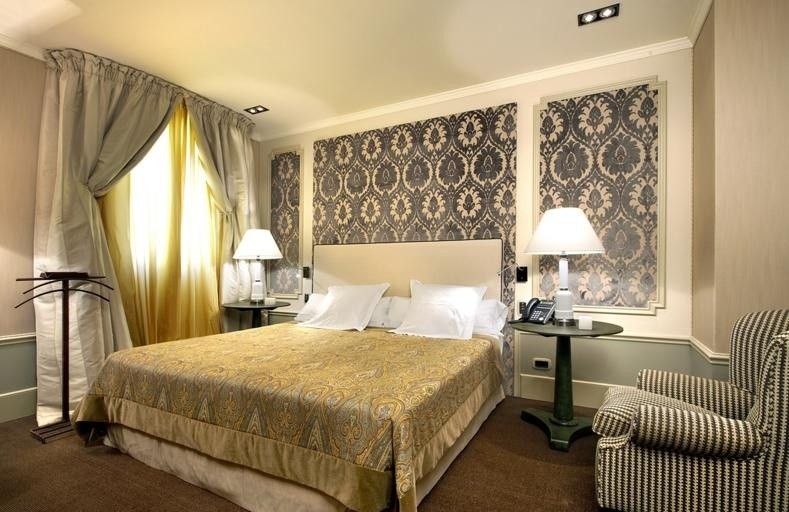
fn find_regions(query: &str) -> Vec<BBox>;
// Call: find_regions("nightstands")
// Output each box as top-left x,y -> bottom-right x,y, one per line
268,310 -> 299,325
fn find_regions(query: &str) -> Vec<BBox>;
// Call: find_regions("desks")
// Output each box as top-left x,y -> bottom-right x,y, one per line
510,319 -> 623,454
221,301 -> 291,328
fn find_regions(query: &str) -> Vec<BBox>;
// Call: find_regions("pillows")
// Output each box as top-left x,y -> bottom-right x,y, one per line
385,279 -> 489,340
293,292 -> 391,328
293,282 -> 390,333
383,295 -> 509,340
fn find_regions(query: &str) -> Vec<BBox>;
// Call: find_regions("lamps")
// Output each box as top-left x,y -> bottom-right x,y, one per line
232,229 -> 284,304
522,207 -> 605,327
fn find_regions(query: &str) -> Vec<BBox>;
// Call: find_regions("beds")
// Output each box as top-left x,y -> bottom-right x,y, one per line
71,238 -> 512,512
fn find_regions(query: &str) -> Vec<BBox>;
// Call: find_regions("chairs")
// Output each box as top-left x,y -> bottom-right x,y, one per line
591,307 -> 789,512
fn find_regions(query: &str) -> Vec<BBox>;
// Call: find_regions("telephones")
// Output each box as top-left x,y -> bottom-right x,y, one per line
522,298 -> 556,325
